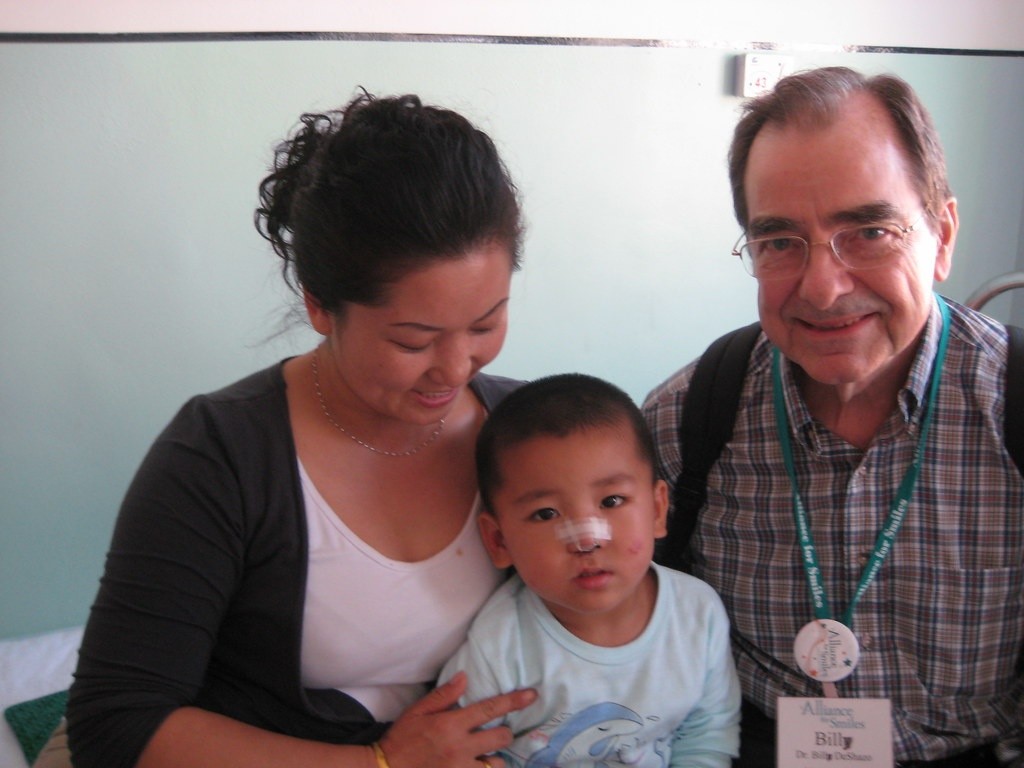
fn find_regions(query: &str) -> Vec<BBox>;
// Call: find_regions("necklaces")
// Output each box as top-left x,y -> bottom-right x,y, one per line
313,345 -> 447,458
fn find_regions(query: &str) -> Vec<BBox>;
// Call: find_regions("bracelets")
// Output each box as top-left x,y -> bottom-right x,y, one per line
373,742 -> 390,768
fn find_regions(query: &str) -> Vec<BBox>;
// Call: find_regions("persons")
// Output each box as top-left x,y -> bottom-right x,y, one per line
638,68 -> 1024,767
436,371 -> 744,768
61,82 -> 538,768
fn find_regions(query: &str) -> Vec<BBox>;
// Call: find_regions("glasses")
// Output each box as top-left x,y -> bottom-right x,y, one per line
730,196 -> 937,282
